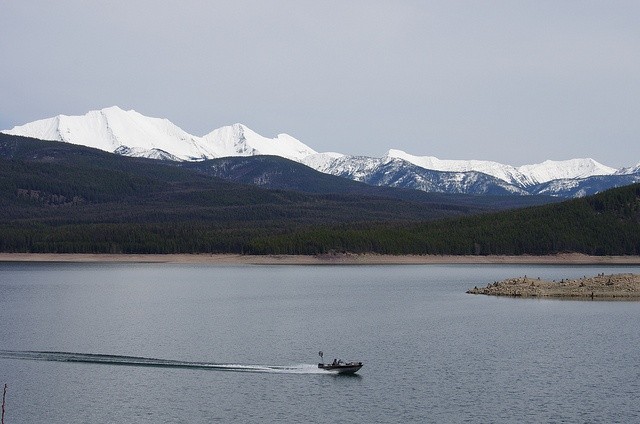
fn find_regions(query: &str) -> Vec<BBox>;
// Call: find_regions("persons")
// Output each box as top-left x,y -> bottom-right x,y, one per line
338,358 -> 343,364
333,358 -> 337,364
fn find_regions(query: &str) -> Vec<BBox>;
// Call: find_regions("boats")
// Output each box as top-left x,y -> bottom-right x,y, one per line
318,351 -> 364,374
